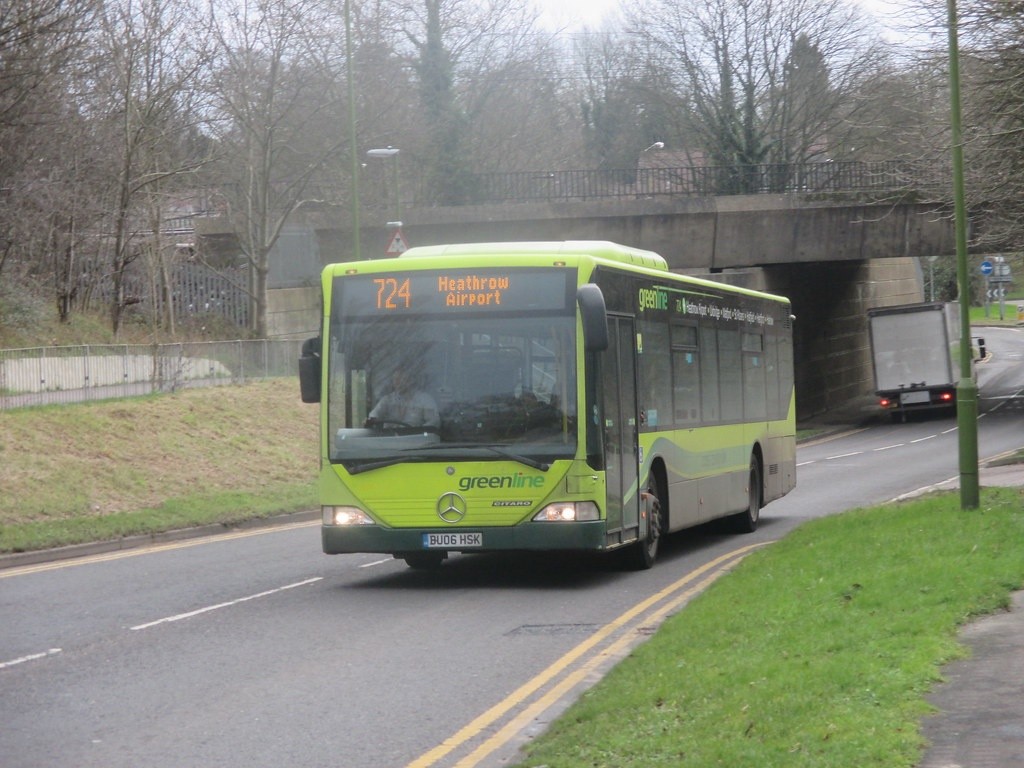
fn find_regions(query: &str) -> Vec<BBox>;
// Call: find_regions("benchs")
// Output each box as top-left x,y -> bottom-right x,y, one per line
469,344 -> 523,392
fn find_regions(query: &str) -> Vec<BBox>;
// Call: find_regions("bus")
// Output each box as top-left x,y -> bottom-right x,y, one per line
299,238 -> 798,571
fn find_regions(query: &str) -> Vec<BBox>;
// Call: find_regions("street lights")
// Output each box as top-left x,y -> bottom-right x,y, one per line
637,139 -> 669,192
367,148 -> 402,228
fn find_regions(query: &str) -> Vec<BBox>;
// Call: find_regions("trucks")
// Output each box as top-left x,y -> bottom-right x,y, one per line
868,302 -> 986,420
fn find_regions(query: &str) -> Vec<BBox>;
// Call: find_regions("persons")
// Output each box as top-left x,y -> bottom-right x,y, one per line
362,368 -> 441,432
